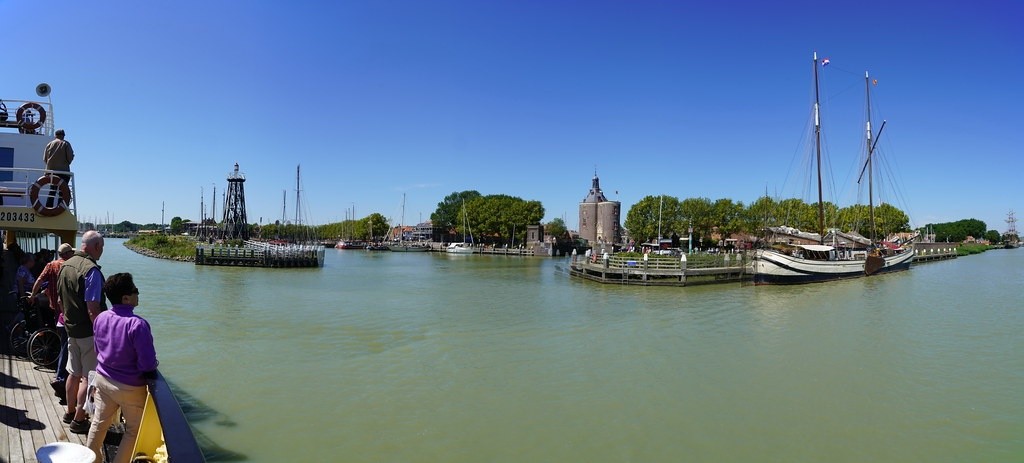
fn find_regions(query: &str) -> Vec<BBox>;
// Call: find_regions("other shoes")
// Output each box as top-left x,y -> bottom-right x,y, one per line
63,411 -> 89,424
70,418 -> 92,435
49,380 -> 66,403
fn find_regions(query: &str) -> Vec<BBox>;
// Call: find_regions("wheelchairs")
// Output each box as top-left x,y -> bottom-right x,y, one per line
10,291 -> 61,367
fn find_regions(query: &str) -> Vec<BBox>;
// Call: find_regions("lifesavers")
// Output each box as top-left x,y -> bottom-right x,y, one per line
30,174 -> 70,217
592,254 -> 597,262
16,103 -> 46,131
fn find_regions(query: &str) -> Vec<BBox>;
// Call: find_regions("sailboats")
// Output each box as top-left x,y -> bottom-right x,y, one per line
335,203 -> 391,250
445,198 -> 475,254
751,50 -> 915,284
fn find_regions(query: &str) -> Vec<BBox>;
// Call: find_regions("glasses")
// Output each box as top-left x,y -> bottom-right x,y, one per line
124,288 -> 139,296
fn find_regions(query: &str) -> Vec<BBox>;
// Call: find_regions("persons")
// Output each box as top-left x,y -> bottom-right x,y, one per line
43,130 -> 75,208
85,272 -> 158,463
0,230 -> 107,436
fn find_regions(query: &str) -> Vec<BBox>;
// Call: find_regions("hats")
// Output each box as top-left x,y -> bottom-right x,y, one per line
58,243 -> 78,254
55,129 -> 65,136
